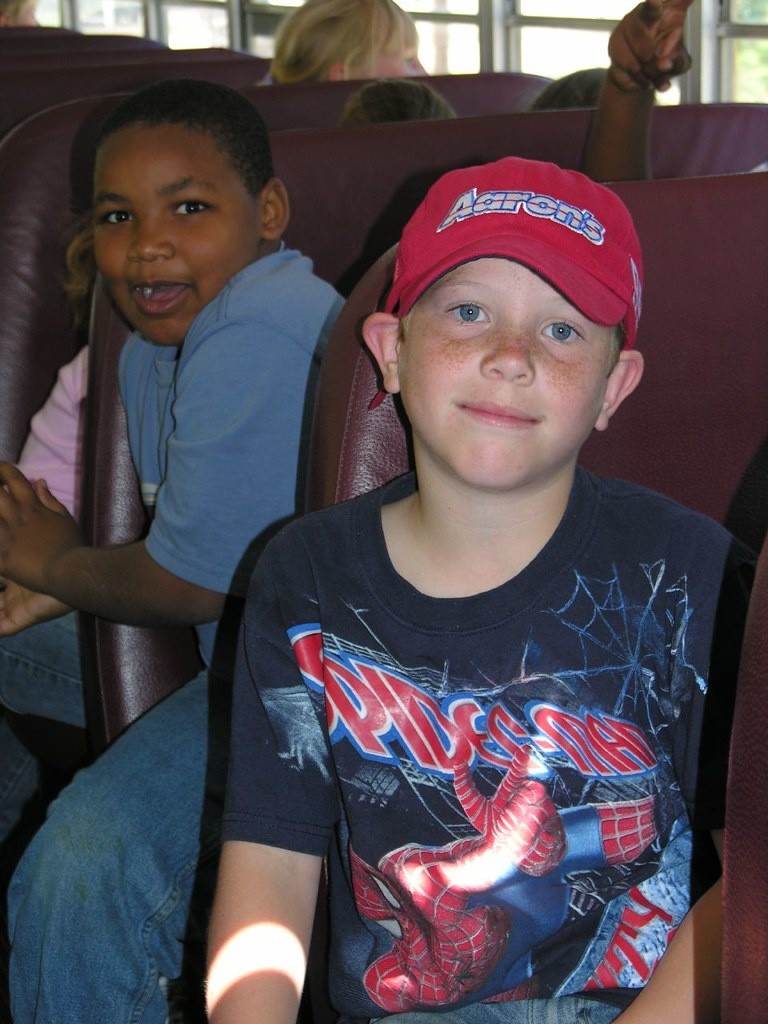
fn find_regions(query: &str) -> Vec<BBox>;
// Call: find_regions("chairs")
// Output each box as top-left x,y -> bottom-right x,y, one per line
0,26 -> 768,1024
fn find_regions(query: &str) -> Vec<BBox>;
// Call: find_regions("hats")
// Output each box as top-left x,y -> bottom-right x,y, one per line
368,156 -> 644,413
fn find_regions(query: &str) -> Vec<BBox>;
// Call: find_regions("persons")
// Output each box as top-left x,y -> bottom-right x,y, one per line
0,77 -> 348,1024
335,80 -> 455,127
269,0 -> 428,83
0,0 -> 44,29
203,155 -> 759,1024
527,70 -> 611,109
572,0 -> 696,183
